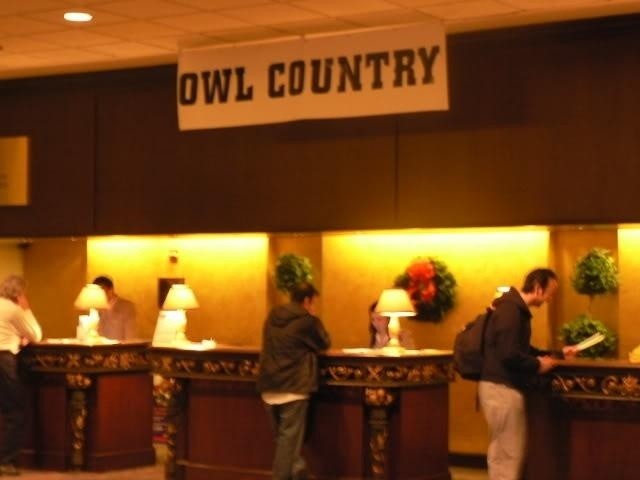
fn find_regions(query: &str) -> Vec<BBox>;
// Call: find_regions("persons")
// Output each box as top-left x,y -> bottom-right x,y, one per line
94,276 -> 138,344
367,302 -> 416,349
255,280 -> 330,480
1,272 -> 43,475
477,269 -> 579,478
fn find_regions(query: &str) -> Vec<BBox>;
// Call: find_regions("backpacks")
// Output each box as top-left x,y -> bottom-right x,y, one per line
452,306 -> 494,383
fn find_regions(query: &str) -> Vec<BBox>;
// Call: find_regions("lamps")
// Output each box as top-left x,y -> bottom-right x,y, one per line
162,283 -> 199,349
73,283 -> 110,345
373,288 -> 418,357
